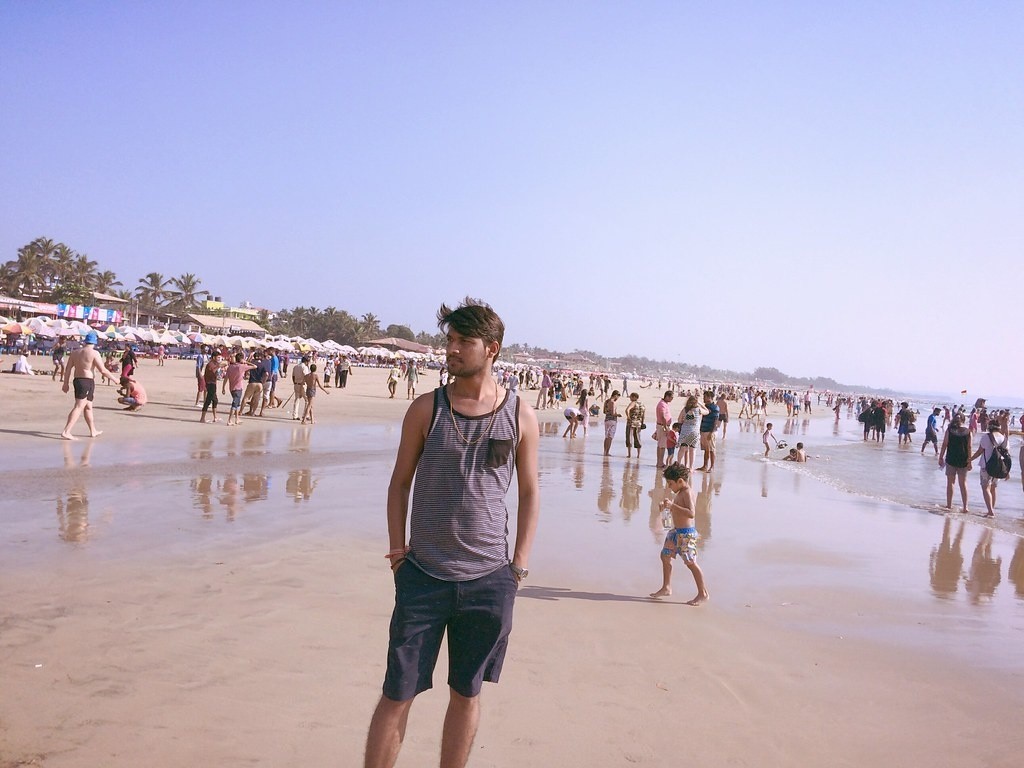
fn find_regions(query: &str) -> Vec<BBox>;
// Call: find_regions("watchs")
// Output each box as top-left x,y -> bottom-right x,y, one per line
508,563 -> 529,582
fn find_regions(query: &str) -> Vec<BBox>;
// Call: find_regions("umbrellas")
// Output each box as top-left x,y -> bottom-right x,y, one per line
0,314 -> 521,369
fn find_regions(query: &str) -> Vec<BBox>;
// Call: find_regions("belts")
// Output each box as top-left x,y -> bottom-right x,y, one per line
294,382 -> 305,385
657,423 -> 670,426
260,380 -> 271,383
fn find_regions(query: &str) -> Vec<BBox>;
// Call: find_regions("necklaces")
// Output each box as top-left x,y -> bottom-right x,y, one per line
450,377 -> 498,445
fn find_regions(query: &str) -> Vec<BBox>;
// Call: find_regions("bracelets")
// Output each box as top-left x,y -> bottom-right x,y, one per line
390,558 -> 405,569
384,545 -> 412,558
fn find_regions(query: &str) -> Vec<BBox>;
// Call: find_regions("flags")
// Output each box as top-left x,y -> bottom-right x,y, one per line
961,390 -> 966,394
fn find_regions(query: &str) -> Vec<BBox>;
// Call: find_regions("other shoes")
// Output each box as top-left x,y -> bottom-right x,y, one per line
293,417 -> 300,420
302,417 -> 310,420
195,404 -> 203,408
208,405 -> 212,408
277,400 -> 282,407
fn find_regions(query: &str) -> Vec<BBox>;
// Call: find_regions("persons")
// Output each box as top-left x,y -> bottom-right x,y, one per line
650,461 -> 709,605
365,295 -> 539,768
0,332 -> 455,440
491,366 -> 1024,517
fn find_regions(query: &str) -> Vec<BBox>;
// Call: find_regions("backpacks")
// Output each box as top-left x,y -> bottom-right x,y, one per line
984,433 -> 1012,479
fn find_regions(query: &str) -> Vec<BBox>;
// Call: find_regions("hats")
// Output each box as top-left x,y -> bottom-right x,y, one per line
80,331 -> 98,345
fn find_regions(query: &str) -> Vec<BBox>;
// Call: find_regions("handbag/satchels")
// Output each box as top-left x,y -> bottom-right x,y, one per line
908,421 -> 916,433
858,411 -> 866,422
869,413 -> 877,425
